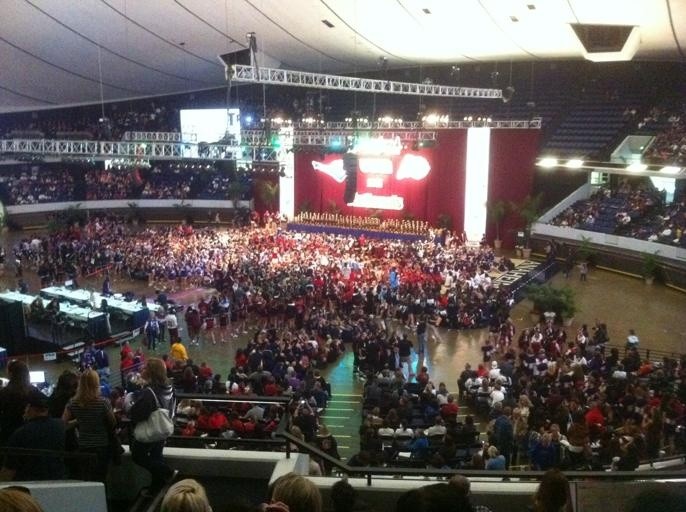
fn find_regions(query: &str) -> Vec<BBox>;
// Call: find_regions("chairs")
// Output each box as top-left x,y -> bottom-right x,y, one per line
360,380 -> 471,475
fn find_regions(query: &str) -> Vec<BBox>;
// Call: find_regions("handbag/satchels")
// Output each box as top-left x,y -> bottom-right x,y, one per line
133,386 -> 175,444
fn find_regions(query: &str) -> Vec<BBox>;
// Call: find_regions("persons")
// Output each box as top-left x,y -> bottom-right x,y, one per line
2,87 -> 684,512
